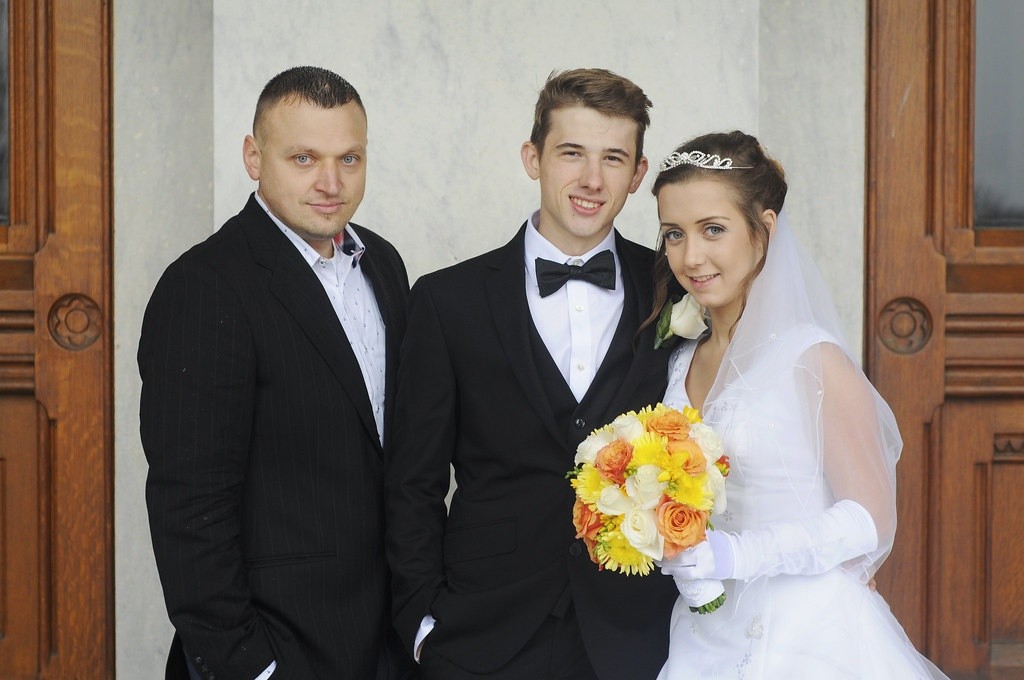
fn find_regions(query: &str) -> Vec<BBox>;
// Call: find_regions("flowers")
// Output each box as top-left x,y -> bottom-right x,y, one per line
569,400 -> 734,614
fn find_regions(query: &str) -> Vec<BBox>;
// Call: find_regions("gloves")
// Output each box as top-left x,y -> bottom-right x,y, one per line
661,527 -> 738,579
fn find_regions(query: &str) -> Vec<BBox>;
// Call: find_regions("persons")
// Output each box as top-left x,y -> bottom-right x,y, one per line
639,131 -> 951,679
386,67 -> 689,679
136,67 -> 411,680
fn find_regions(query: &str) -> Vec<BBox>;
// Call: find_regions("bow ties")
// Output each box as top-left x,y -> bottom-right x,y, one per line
535,249 -> 616,298
